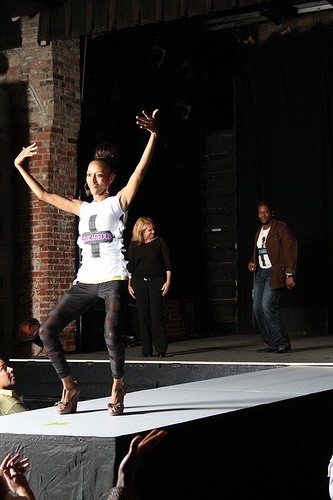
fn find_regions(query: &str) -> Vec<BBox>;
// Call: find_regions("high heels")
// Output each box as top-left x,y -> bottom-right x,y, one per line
108,383 -> 125,416
57,381 -> 80,414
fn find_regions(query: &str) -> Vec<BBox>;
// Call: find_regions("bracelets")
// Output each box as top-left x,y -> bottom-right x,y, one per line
285,275 -> 292,279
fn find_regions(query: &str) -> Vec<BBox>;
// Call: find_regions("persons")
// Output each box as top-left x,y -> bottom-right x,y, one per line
247,199 -> 297,353
14,108 -> 158,416
0,352 -> 167,500
127,217 -> 173,357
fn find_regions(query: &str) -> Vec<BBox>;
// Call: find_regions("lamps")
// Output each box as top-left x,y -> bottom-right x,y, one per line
17,318 -> 48,359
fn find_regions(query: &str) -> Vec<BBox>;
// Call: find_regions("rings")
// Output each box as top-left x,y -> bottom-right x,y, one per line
252,268 -> 254,271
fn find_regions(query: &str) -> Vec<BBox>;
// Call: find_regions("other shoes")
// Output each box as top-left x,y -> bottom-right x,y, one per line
158,352 -> 166,357
140,353 -> 153,357
275,345 -> 291,354
256,348 -> 278,353
